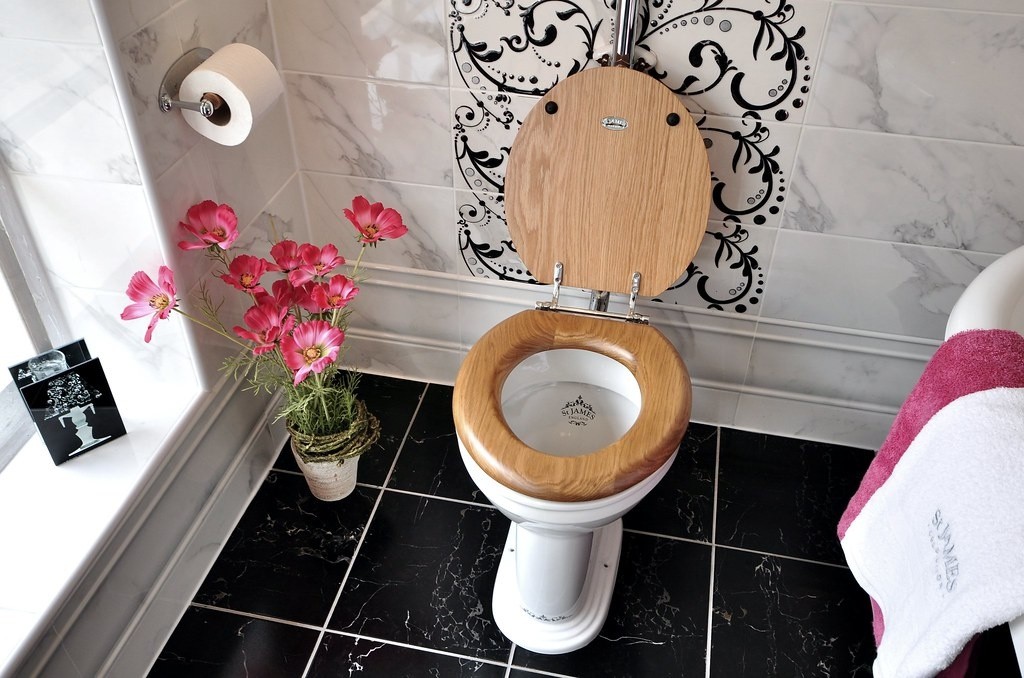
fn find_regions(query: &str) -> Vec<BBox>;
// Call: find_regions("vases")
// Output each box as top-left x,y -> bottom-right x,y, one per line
291,436 -> 360,502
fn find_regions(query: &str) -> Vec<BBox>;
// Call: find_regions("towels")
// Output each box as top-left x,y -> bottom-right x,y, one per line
836,328 -> 1024,678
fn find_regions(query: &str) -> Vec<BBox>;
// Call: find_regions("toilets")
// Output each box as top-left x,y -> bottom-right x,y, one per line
452,65 -> 713,656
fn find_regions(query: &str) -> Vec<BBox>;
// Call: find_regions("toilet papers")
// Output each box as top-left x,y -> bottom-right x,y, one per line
178,42 -> 282,147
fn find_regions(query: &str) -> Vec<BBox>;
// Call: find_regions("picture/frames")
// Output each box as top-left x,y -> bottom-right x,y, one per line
19,357 -> 127,466
9,337 -> 92,426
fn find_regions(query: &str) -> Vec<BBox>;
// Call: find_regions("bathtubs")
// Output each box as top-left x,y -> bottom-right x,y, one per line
944,244 -> 1020,674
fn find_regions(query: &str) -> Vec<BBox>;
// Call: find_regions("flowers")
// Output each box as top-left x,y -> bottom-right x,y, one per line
119,195 -> 408,433
